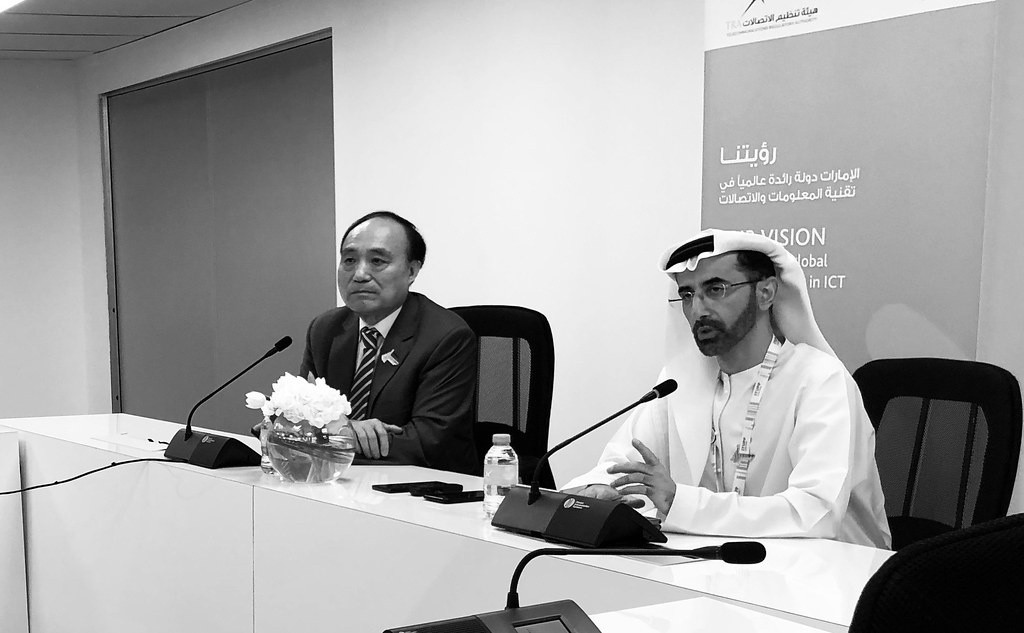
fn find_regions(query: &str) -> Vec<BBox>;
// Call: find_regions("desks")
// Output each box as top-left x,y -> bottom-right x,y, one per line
1,413 -> 898,632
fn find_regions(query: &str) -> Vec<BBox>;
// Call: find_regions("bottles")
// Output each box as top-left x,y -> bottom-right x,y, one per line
483,434 -> 519,520
260,397 -> 281,476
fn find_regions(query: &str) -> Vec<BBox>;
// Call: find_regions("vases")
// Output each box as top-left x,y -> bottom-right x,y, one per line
267,412 -> 354,484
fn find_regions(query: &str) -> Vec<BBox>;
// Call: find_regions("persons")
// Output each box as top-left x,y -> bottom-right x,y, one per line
299,211 -> 477,476
559,229 -> 892,552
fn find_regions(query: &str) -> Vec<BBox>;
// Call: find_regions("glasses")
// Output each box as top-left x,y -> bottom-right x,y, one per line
668,279 -> 758,309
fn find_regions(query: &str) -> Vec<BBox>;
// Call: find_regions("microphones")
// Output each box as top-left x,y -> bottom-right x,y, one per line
385,542 -> 767,633
164,336 -> 293,469
491,379 -> 677,548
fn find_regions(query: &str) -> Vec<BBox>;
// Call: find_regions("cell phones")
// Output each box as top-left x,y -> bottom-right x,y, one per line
372,481 -> 485,504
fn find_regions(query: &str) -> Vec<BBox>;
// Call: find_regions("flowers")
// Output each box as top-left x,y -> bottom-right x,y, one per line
245,372 -> 352,482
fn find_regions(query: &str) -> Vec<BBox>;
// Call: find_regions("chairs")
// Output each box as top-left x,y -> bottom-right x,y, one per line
447,305 -> 557,489
850,513 -> 1023,633
851,357 -> 1024,552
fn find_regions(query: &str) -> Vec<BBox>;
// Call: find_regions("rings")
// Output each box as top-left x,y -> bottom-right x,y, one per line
644,485 -> 648,495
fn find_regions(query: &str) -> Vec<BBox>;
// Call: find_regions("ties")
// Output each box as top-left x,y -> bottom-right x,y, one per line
348,327 -> 380,420
